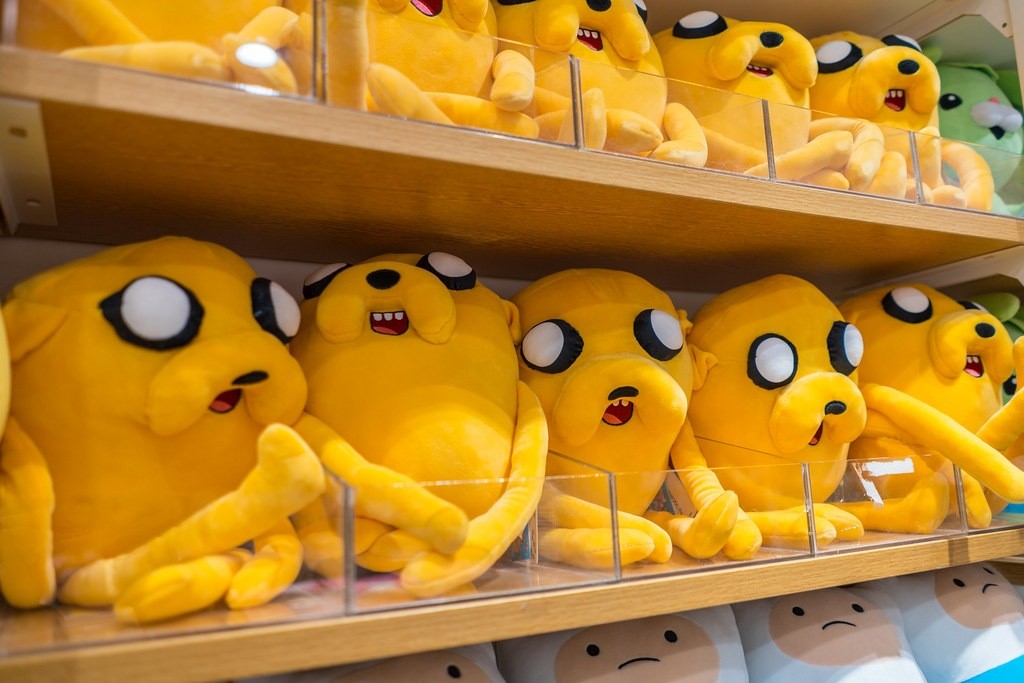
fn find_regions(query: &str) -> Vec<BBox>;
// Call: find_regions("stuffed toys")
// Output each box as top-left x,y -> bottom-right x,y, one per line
0,236 -> 1023,683
0,0 -> 1021,219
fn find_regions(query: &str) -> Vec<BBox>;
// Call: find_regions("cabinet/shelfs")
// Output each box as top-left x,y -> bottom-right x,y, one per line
0,0 -> 1024,683
